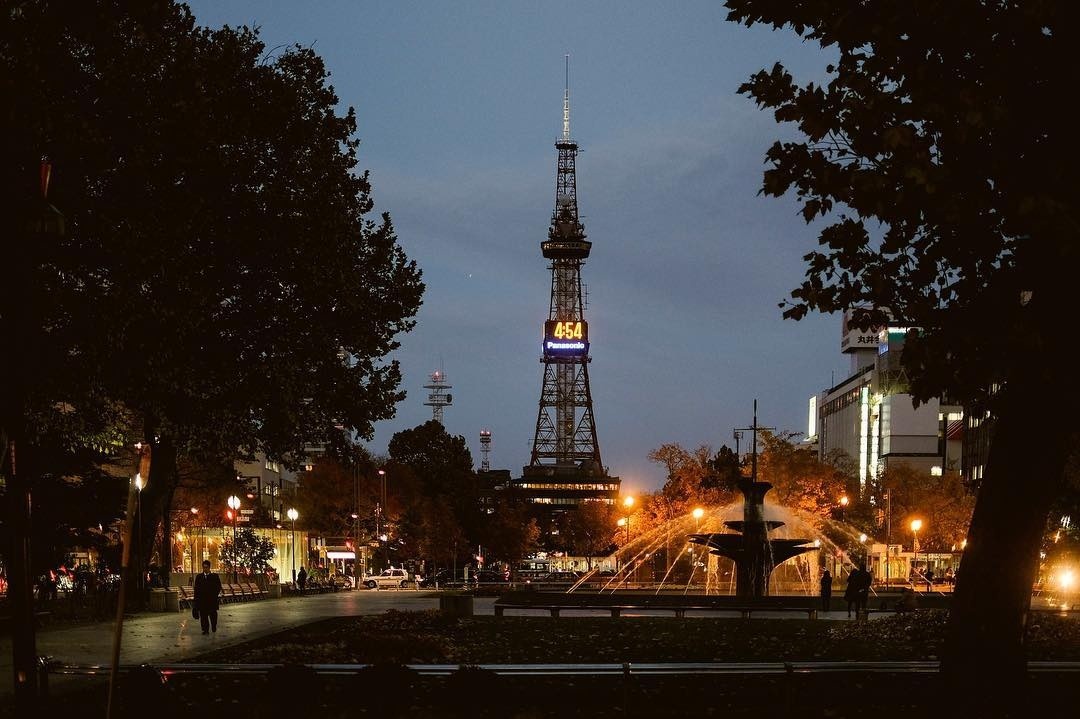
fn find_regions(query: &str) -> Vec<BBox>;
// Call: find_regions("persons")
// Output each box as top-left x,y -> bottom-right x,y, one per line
910,567 -> 957,592
843,563 -> 872,619
192,560 -> 222,635
328,574 -> 338,593
307,576 -> 323,591
297,566 -> 307,595
820,570 -> 833,612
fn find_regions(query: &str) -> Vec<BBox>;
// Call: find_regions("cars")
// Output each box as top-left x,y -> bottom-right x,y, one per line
362,568 -> 422,588
472,570 -> 614,582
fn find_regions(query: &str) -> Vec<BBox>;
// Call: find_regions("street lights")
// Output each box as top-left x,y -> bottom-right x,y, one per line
236,475 -> 263,529
692,507 -> 705,562
911,519 -> 921,587
623,496 -> 634,582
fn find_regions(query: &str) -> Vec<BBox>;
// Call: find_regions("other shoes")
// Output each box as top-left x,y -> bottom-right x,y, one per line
212,625 -> 217,632
203,629 -> 209,635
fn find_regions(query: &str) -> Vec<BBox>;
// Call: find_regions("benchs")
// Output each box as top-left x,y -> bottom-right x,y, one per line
858,605 -> 916,620
489,603 -> 820,618
285,580 -> 321,596
155,582 -> 270,611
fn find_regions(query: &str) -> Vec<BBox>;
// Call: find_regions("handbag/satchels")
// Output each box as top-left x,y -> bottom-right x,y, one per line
192,608 -> 199,620
